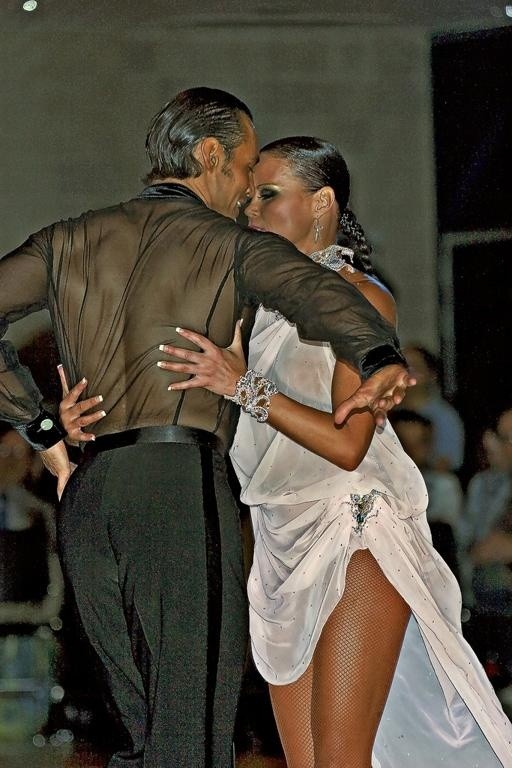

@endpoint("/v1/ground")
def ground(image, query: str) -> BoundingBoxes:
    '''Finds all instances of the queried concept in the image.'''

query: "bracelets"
[225,368,276,424]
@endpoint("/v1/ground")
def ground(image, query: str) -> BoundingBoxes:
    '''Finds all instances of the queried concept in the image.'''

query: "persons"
[1,85,512,768]
[58,135,512,767]
[388,342,512,720]
[0,420,66,635]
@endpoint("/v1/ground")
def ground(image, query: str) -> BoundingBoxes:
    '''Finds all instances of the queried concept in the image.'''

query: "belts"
[83,425,225,456]
